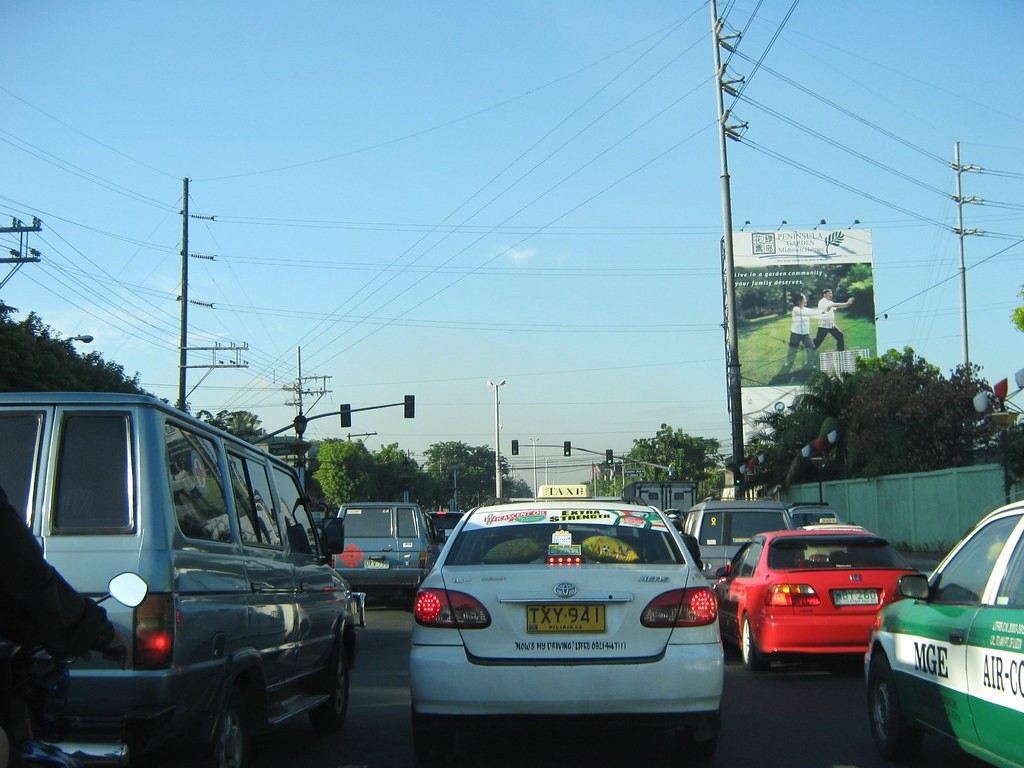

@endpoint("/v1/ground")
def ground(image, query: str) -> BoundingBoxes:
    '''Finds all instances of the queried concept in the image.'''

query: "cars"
[406,484,729,739]
[684,499,1024,768]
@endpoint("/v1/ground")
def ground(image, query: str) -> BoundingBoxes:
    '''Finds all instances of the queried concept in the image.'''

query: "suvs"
[0,390,368,768]
[328,501,466,607]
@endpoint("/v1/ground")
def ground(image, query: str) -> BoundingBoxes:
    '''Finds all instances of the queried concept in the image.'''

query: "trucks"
[624,480,698,515]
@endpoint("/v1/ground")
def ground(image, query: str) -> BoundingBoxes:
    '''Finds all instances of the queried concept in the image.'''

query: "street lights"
[490,381,507,507]
[529,437,540,500]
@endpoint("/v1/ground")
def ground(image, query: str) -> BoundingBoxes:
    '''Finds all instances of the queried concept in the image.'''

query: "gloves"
[45,597,116,661]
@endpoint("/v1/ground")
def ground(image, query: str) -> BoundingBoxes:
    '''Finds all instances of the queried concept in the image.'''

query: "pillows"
[581,536,639,563]
[483,538,542,564]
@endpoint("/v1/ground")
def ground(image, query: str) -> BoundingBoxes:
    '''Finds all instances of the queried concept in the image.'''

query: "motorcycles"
[8,563,149,754]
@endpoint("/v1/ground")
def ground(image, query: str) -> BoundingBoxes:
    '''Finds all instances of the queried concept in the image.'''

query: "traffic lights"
[511,439,519,455]
[606,450,613,464]
[564,442,571,456]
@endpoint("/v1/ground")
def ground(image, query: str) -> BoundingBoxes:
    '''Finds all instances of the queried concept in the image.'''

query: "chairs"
[829,551,845,566]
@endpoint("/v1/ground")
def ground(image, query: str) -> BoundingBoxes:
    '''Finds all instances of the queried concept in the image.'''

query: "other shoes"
[785,361,793,367]
[804,362,817,369]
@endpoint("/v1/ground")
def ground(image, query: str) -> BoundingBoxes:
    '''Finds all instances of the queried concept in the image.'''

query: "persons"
[813,289,855,353]
[0,486,130,768]
[786,294,816,369]
